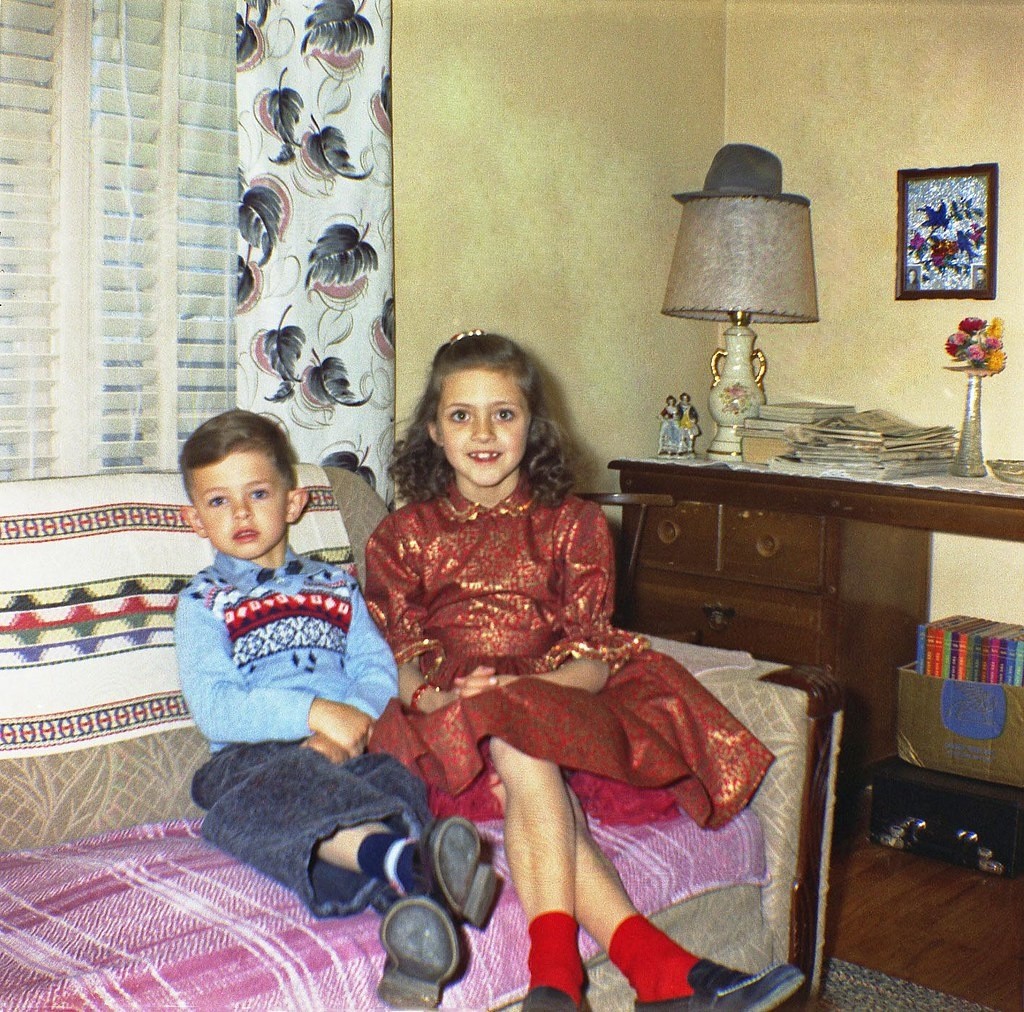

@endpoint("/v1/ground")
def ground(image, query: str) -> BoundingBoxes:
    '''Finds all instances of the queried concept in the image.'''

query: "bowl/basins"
[987,460,1024,484]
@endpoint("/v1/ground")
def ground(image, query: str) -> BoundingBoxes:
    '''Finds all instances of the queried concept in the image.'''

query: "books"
[735,400,960,479]
[916,615,1024,687]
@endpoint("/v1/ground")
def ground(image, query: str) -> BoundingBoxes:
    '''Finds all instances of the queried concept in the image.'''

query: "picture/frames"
[895,164,997,300]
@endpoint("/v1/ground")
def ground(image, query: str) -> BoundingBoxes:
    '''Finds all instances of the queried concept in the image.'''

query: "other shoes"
[634,958,807,1012]
[376,894,462,1012]
[416,815,498,929]
[520,967,589,1012]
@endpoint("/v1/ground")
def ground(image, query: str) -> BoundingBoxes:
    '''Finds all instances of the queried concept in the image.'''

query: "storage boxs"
[895,660,1024,788]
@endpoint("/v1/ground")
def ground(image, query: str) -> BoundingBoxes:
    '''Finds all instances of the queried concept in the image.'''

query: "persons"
[175,409,497,1012]
[658,393,700,455]
[361,330,807,1012]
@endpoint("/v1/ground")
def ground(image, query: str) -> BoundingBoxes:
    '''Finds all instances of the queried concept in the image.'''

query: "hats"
[672,143,811,208]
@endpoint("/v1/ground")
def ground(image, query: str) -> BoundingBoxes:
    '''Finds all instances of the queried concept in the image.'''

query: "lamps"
[662,197,818,459]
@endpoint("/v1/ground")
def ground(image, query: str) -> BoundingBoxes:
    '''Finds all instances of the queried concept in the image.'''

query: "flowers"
[944,317,1006,371]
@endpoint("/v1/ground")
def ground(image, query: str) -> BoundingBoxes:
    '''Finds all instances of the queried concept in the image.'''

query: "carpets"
[820,958,991,1012]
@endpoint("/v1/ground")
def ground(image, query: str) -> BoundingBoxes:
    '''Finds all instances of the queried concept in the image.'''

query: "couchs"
[0,464,845,1012]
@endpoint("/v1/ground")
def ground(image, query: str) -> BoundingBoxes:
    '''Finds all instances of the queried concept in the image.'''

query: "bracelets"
[410,682,437,709]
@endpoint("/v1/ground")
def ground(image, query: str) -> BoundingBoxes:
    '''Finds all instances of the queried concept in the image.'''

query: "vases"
[943,365,992,478]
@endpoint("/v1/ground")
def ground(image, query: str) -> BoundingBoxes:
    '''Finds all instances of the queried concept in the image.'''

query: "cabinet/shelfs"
[608,460,1024,807]
